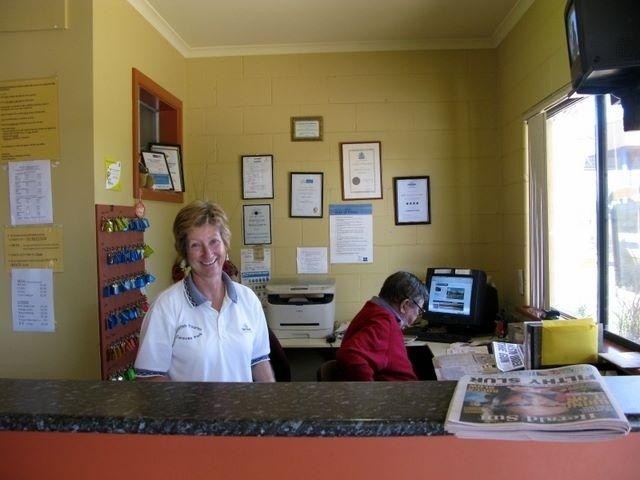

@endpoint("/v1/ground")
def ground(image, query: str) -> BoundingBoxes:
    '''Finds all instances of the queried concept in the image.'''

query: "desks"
[275,325,427,359]
[428,330,524,380]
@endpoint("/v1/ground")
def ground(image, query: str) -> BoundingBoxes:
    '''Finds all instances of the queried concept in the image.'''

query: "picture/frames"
[149,143,185,192]
[290,116,323,142]
[340,141,383,200]
[393,175,432,226]
[142,149,176,190]
[289,171,324,217]
[243,203,271,246]
[240,154,275,200]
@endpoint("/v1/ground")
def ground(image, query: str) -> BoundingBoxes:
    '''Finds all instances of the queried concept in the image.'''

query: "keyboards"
[414,332,468,341]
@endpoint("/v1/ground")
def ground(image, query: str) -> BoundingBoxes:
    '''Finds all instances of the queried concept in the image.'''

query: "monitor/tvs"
[423,267,499,330]
[561,0,640,94]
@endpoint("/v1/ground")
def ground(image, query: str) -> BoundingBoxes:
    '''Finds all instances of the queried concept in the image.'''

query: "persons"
[132,199,276,383]
[222,260,292,383]
[336,270,430,381]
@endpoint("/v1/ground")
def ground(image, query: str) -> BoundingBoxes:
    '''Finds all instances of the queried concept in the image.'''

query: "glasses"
[401,297,426,315]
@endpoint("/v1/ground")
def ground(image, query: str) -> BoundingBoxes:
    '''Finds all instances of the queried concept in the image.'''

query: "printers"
[265,279,336,340]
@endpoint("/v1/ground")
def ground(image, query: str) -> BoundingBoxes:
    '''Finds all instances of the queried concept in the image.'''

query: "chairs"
[316,360,338,382]
